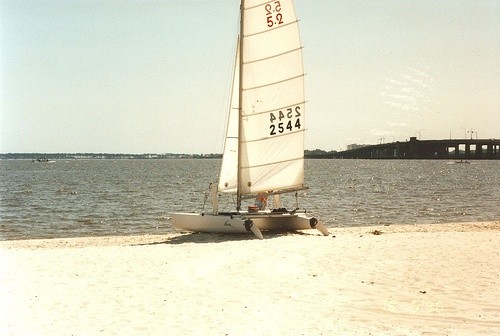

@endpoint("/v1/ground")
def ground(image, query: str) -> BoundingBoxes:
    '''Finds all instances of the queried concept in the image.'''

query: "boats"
[30,158,56,163]
[455,160,470,164]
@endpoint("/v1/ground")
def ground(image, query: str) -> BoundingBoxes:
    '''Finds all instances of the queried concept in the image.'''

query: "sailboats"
[167,0,332,239]
